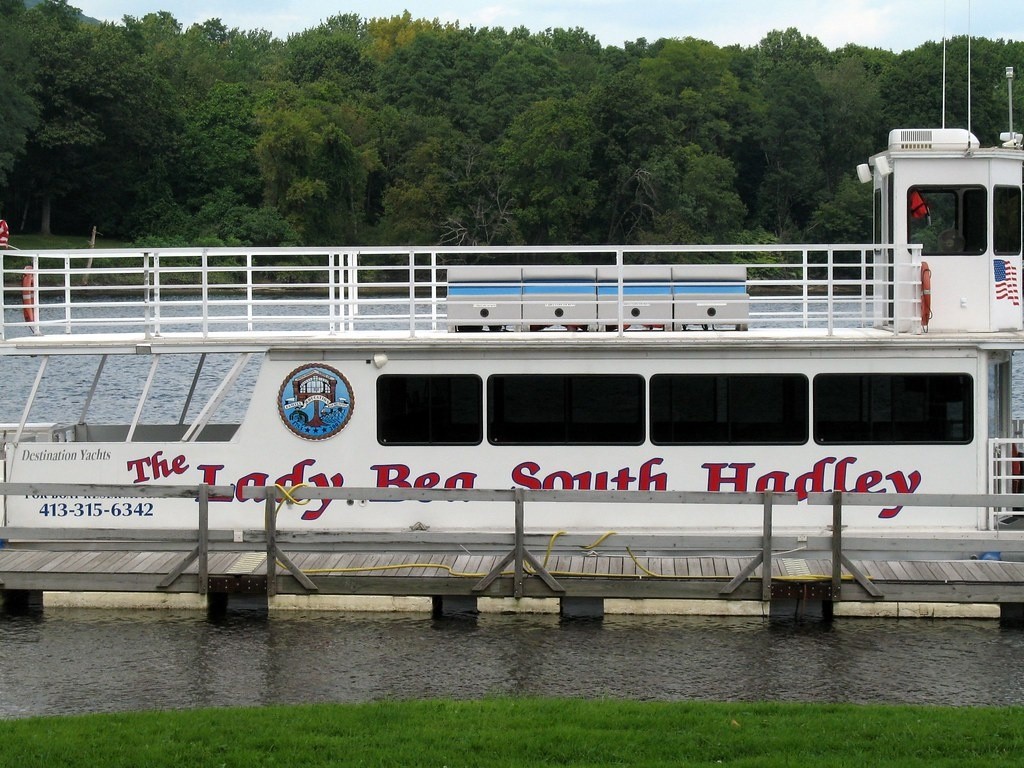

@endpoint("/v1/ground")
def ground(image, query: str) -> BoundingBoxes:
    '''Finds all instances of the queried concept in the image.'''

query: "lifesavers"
[20,265,34,322]
[921,260,931,326]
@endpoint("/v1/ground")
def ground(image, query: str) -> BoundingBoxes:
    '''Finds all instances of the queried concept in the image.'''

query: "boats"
[0,2,1024,559]
